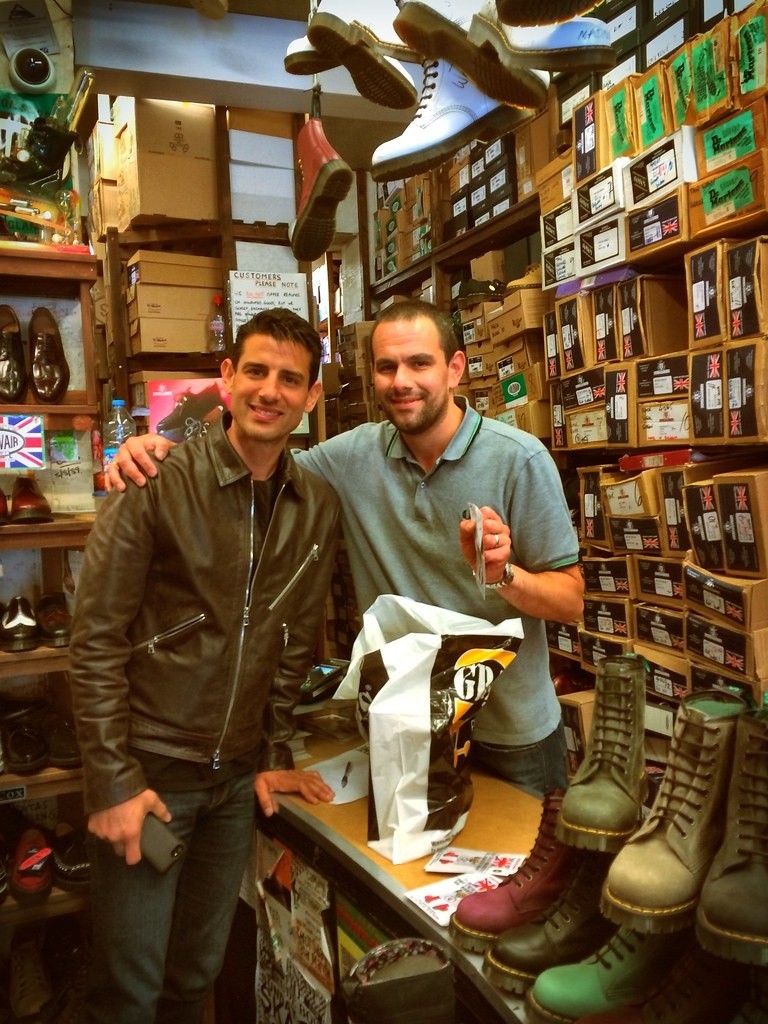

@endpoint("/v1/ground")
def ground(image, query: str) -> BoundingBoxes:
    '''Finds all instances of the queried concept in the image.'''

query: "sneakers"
[10,827,53,901]
[9,928,58,1024]
[0,596,39,651]
[506,262,542,293]
[52,821,93,890]
[457,279,504,308]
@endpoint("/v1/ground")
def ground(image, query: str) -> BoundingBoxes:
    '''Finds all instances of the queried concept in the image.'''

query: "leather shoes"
[29,307,70,405]
[0,486,10,525]
[10,478,53,522]
[37,706,82,766]
[0,700,50,771]
[0,304,28,404]
[284,0,620,109]
[37,592,71,647]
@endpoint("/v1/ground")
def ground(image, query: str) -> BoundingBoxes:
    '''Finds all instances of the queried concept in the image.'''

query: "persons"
[105,300,586,797]
[68,305,351,1024]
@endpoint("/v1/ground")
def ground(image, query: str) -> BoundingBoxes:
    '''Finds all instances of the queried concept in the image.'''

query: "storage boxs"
[0,0,768,765]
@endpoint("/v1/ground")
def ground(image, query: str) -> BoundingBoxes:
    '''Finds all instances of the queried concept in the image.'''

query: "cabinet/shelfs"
[104,103,319,449]
[0,249,106,929]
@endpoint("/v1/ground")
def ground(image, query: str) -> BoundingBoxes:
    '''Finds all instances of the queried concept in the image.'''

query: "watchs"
[472,561,515,589]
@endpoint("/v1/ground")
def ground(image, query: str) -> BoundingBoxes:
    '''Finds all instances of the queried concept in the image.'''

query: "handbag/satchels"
[331,594,525,864]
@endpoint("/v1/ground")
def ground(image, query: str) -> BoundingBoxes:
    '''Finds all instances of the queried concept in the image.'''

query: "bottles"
[104,398,137,481]
[208,294,225,354]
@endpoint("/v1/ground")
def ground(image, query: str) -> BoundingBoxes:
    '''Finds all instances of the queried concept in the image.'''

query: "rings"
[494,533,499,548]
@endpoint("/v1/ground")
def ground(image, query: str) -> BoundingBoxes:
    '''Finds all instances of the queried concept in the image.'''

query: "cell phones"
[136,814,188,877]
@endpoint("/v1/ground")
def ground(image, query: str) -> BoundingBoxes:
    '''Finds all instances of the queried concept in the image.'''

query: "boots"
[291,119,354,262]
[0,117,78,200]
[370,57,538,182]
[449,786,768,1024]
[556,656,768,966]
[344,937,455,1024]
